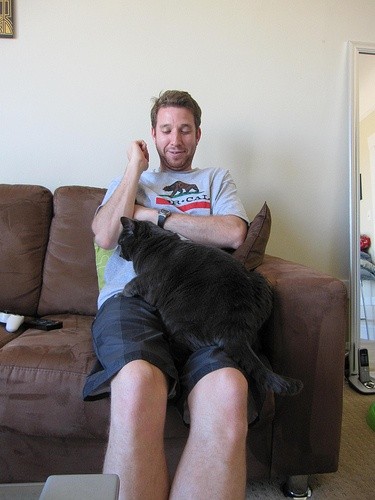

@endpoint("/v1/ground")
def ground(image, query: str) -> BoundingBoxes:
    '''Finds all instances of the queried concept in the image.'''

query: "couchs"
[0,184,348,500]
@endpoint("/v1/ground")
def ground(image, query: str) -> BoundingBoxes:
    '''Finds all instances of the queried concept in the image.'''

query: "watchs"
[157,208,172,228]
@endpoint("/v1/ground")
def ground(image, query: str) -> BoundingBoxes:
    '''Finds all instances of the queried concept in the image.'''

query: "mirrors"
[348,40,375,376]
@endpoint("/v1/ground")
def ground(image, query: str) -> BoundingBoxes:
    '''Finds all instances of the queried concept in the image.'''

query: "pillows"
[233,201,272,272]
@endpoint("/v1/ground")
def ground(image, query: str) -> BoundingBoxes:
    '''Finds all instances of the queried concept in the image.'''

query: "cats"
[118,217,306,397]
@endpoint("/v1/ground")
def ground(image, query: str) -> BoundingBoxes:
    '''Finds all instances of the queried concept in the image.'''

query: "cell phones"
[22,318,62,332]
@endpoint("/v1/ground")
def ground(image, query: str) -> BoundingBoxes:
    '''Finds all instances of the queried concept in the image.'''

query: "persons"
[81,90,250,500]
